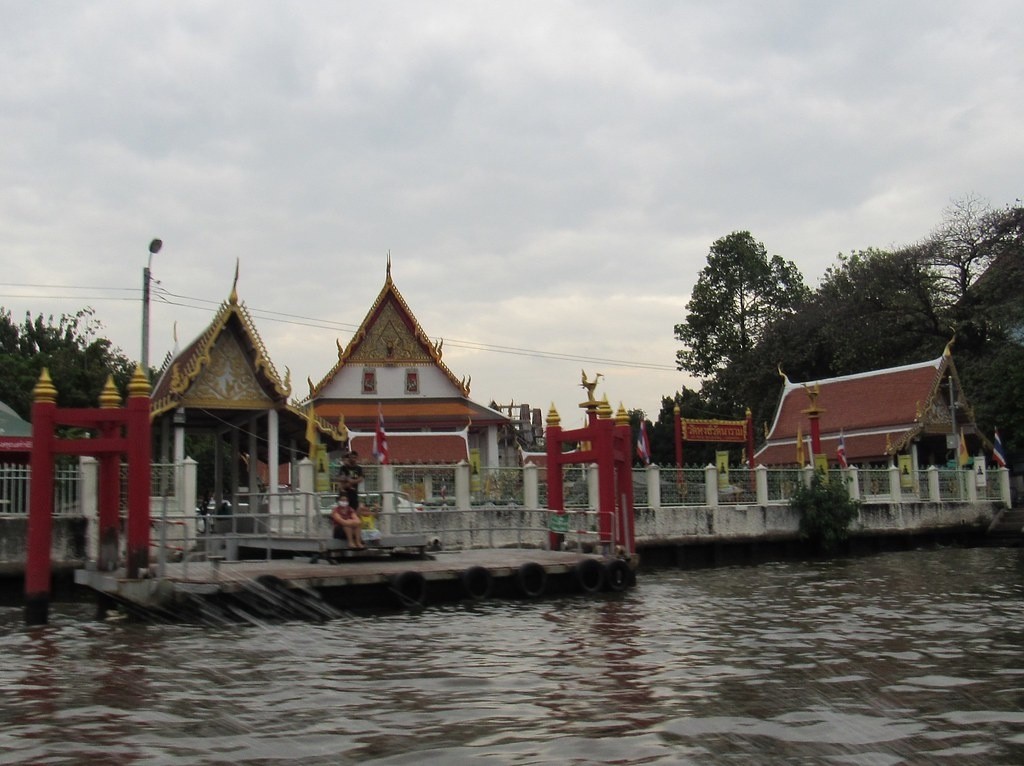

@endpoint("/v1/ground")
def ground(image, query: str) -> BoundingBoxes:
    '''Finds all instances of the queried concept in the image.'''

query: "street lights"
[141,239,163,378]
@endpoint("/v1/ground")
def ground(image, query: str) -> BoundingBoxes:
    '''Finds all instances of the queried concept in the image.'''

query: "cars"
[329,493,424,513]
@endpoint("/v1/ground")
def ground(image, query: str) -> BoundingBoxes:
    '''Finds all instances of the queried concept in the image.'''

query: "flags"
[796,425,804,463]
[441,477,447,498]
[636,416,652,465]
[837,432,847,468]
[959,430,969,465]
[993,428,1006,466]
[373,406,390,464]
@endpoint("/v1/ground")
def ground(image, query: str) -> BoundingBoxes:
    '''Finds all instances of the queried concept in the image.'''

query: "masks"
[338,501,347,505]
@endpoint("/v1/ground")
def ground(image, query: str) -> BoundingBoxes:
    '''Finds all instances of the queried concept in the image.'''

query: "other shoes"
[348,544,364,548]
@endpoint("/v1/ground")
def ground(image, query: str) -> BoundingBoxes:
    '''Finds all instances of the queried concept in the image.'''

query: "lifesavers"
[247,574,291,617]
[515,561,548,598]
[463,566,492,601]
[390,570,428,608]
[575,558,605,594]
[606,558,630,592]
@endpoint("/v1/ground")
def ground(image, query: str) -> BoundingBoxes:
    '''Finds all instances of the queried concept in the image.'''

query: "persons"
[331,450,374,548]
